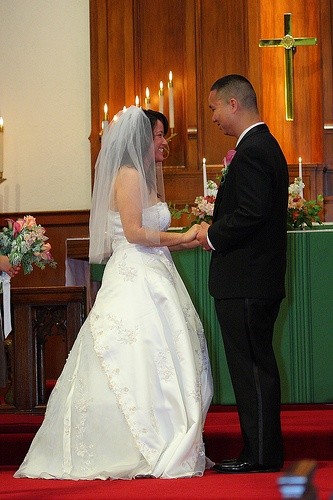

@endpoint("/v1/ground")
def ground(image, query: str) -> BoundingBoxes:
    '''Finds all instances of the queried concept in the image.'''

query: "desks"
[65,226,333,405]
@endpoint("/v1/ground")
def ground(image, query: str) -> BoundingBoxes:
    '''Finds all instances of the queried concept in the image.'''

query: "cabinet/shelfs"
[88,0,333,222]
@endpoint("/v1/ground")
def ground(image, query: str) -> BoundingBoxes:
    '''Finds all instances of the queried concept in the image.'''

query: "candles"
[157,81,164,115]
[202,157,208,197]
[144,86,151,110]
[135,96,140,108]
[168,70,175,129]
[299,156,303,199]
[102,103,109,128]
[0,118,5,179]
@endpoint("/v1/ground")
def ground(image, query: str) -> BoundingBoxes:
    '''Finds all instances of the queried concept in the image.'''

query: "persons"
[0,255,21,278]
[13,105,214,481]
[196,74,289,473]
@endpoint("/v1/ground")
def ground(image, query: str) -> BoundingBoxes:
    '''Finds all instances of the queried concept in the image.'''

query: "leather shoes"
[212,458,281,472]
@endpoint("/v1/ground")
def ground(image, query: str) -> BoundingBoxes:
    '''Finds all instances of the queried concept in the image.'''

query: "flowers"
[286,177,326,230]
[167,150,236,233]
[0,215,58,293]
